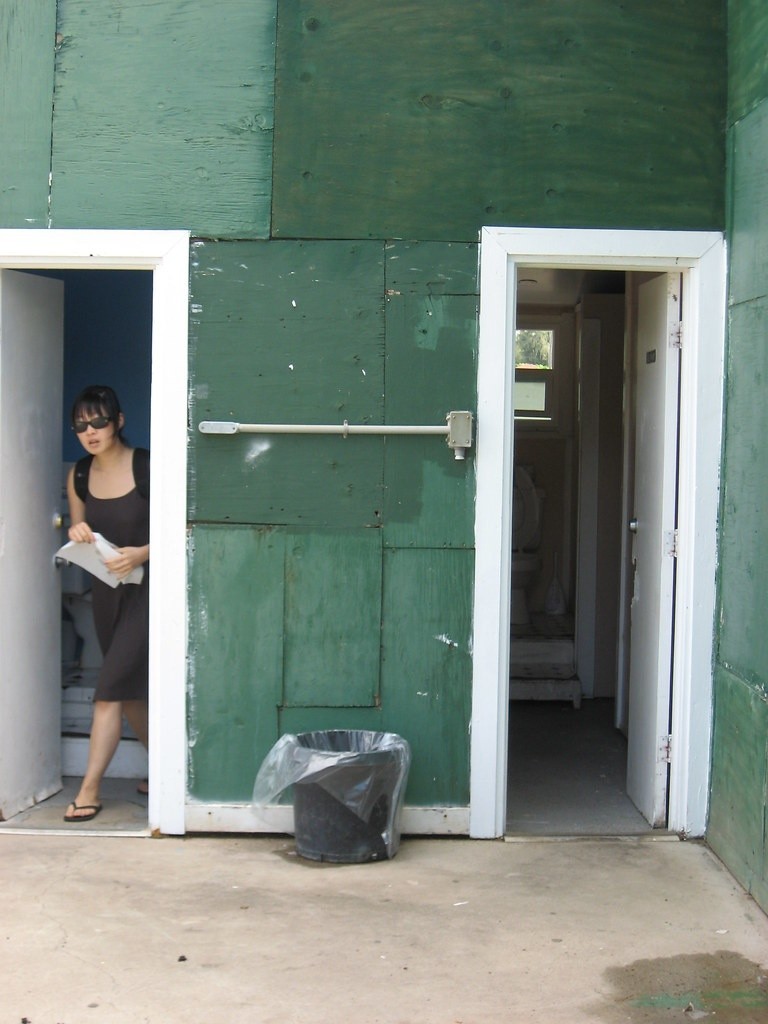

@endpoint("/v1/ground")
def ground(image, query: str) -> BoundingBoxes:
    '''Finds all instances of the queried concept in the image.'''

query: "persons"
[62,384,150,823]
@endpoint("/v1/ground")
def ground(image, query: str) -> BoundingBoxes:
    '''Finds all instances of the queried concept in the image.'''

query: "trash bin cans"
[289,729,407,864]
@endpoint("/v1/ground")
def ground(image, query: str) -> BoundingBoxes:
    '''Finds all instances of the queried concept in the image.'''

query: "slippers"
[137,778,148,795]
[63,802,103,822]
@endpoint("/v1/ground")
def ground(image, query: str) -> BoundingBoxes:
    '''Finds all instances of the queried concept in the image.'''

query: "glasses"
[71,416,114,433]
